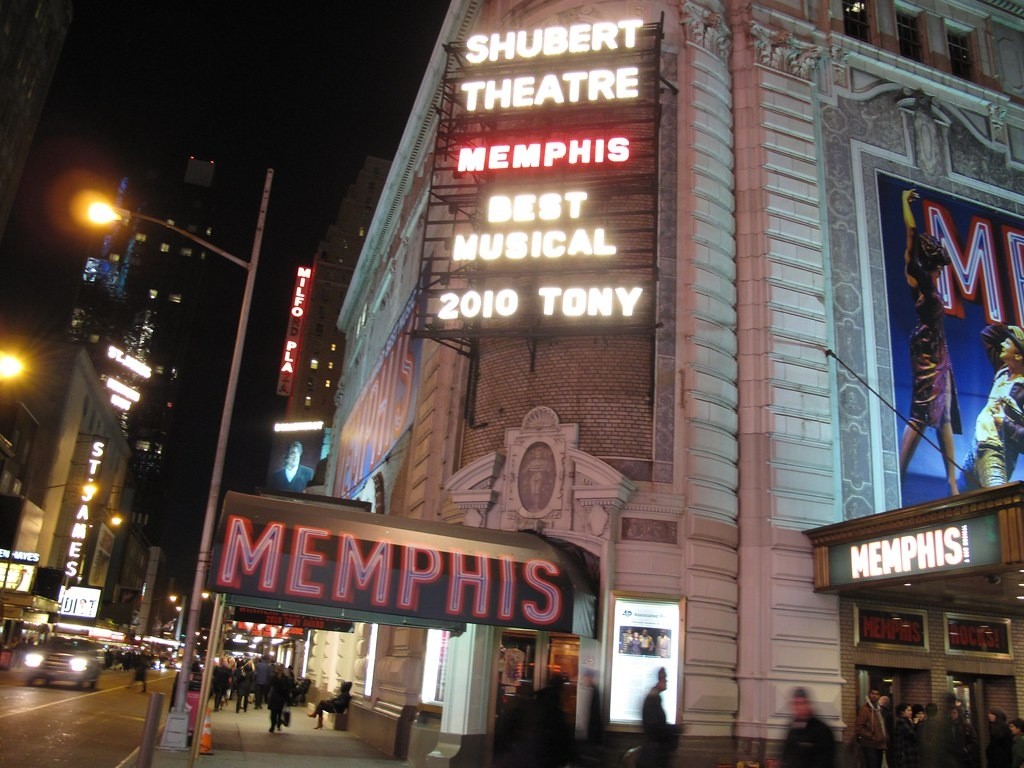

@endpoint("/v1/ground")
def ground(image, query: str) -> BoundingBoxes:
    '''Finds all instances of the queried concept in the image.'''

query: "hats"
[1008,326,1024,350]
[990,705,1008,720]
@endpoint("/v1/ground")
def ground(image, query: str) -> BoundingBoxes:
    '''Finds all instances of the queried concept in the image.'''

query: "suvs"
[24,638,100,690]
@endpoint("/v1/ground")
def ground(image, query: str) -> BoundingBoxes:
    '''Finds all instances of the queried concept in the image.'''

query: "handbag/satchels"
[283,707,290,727]
[237,670,246,682]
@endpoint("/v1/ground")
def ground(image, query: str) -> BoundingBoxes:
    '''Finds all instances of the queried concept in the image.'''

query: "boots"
[307,710,318,718]
[314,716,323,729]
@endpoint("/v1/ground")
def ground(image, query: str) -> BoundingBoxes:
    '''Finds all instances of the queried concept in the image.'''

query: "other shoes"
[277,725,280,731]
[269,728,274,732]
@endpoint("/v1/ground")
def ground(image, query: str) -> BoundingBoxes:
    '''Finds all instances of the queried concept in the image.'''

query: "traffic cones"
[200,708,216,753]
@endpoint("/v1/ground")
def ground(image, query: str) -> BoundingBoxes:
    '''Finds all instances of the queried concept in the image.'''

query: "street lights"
[68,169,280,748]
[168,592,186,641]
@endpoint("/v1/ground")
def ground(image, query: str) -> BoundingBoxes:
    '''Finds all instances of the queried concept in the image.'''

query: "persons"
[780,687,1024,768]
[271,441,314,493]
[0,637,355,733]
[619,628,671,658]
[899,189,1024,498]
[487,667,686,768]
